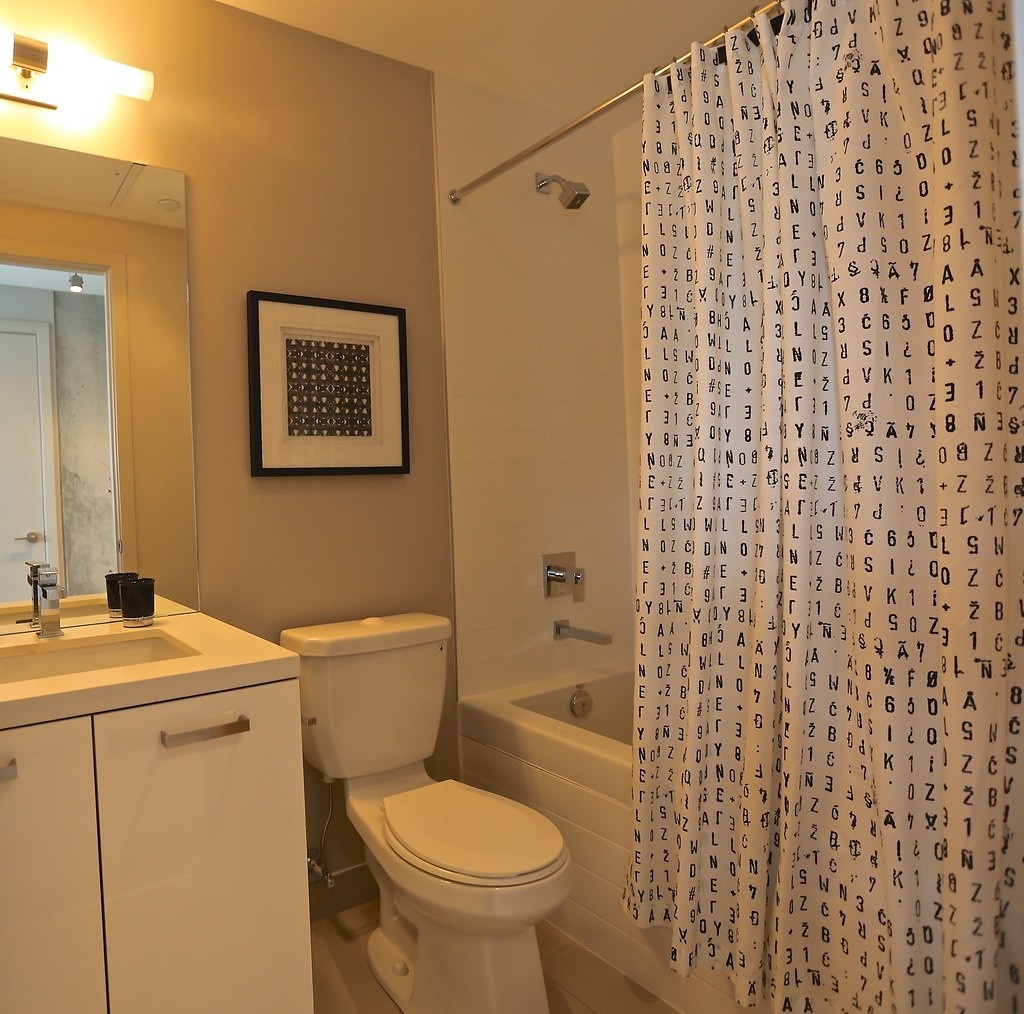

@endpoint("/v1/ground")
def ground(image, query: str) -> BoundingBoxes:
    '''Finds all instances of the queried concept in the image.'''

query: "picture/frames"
[246,289,411,476]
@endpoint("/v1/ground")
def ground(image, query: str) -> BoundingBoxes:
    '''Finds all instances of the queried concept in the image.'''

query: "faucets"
[36,568,66,639]
[552,615,614,649]
[23,561,51,627]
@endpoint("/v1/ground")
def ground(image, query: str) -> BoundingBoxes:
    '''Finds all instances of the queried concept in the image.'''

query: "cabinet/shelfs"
[1,654,317,1012]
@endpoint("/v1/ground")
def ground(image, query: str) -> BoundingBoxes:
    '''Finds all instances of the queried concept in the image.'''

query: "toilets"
[278,611,576,1013]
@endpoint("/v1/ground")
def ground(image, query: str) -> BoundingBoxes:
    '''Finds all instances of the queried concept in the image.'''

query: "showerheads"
[536,171,588,212]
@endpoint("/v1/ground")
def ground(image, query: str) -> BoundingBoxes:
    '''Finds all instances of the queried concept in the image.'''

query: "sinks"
[0,626,205,691]
[1,595,118,626]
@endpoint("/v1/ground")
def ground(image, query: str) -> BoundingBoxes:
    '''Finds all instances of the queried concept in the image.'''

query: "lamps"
[0,24,154,112]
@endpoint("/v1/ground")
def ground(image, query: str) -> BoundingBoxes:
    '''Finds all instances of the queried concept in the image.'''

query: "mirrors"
[0,264,120,603]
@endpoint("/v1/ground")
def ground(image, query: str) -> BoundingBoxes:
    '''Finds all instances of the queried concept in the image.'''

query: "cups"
[118,577,155,628]
[105,572,139,618]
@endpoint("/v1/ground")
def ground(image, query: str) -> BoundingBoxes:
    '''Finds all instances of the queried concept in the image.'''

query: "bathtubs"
[460,666,778,1012]
